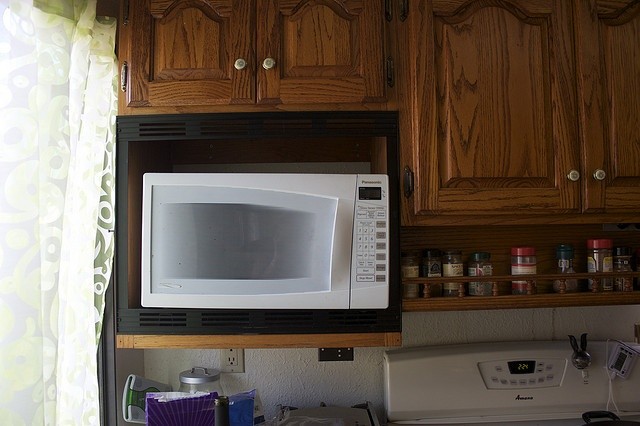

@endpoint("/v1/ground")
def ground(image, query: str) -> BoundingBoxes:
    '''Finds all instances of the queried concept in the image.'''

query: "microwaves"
[141,172,389,311]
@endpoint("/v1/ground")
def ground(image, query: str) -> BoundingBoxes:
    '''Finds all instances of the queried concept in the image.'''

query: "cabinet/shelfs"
[399,3,639,226]
[116,0,399,116]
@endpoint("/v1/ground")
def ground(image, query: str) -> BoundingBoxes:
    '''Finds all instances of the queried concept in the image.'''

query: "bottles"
[177,365,225,397]
[586,238,615,293]
[421,247,444,299]
[510,246,538,296]
[399,256,421,298]
[613,246,634,294]
[468,252,493,296]
[551,241,578,293]
[439,248,467,298]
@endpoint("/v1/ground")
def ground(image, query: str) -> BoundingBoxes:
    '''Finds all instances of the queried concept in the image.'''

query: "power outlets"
[219,348,245,373]
